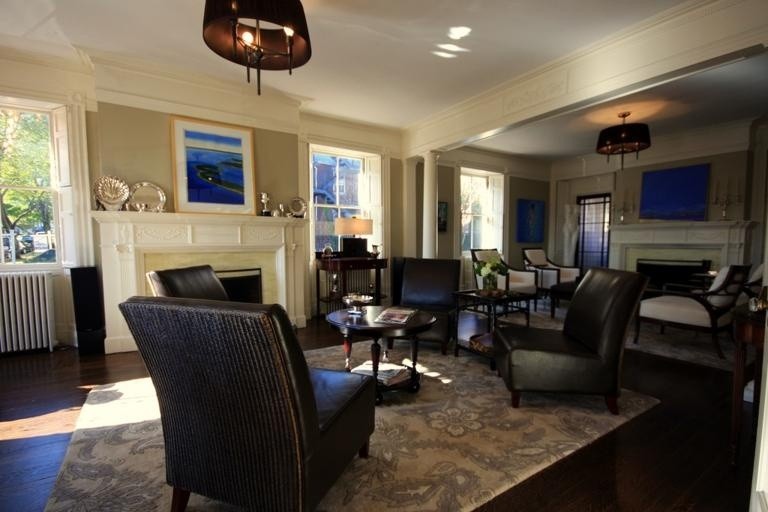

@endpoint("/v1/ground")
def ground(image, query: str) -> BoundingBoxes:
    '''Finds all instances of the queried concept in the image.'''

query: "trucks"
[313,189,357,251]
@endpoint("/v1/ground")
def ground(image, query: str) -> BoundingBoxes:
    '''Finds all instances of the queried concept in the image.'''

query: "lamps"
[596,112,651,172]
[203,1,312,95]
[333,216,373,256]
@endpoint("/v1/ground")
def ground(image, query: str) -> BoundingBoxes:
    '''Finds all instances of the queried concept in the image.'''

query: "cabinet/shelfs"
[315,257,387,330]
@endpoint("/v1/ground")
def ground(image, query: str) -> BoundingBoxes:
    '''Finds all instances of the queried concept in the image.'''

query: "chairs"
[470,247,581,311]
[489,269,650,415]
[388,257,461,356]
[145,262,229,301]
[118,294,379,511]
[633,261,765,360]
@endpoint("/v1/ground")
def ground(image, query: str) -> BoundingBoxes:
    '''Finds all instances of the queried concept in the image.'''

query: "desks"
[728,301,768,466]
[456,290,532,334]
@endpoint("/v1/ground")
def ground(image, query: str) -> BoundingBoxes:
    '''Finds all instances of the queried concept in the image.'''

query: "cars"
[2,226,46,258]
[25,249,56,262]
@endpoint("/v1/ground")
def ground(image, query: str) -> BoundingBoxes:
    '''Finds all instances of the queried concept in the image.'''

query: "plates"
[288,196,307,216]
[128,181,166,213]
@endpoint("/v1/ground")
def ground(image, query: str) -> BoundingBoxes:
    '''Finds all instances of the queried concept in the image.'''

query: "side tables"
[550,281,578,318]
[461,288,542,333]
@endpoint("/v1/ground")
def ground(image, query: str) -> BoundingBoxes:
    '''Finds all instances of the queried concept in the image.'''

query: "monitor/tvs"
[639,162,711,223]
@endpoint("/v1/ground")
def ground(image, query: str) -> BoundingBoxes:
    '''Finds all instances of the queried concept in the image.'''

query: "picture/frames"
[438,201,448,232]
[170,115,257,216]
[515,198,544,244]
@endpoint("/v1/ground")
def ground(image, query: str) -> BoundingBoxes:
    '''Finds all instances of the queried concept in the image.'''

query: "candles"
[612,187,636,210]
[712,174,739,204]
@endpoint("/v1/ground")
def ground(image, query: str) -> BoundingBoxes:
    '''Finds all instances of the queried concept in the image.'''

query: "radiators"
[0,272,59,353]
[319,268,373,315]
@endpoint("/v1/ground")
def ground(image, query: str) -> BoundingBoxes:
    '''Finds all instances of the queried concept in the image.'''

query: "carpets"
[461,295,757,373]
[42,333,661,511]
